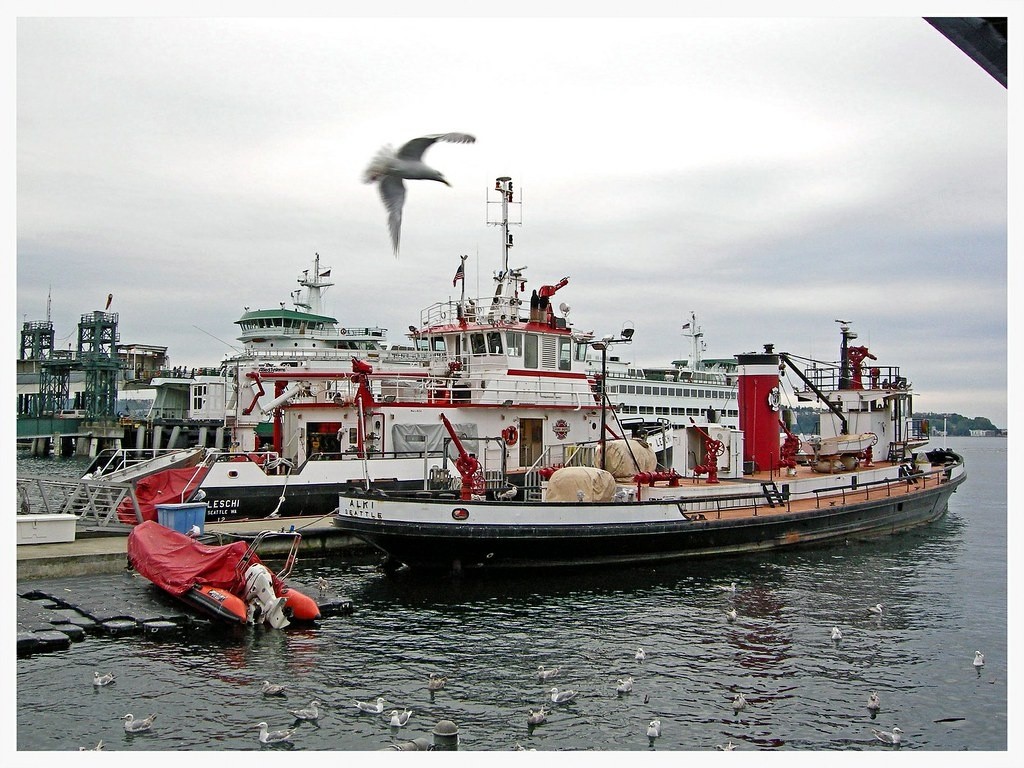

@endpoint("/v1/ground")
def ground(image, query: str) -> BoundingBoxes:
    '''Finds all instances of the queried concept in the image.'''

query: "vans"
[53,407,96,420]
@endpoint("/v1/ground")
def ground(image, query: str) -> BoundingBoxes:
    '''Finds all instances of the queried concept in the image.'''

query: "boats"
[128,520,321,624]
[333,344,968,574]
[76,176,786,527]
[801,434,876,455]
[144,250,739,430]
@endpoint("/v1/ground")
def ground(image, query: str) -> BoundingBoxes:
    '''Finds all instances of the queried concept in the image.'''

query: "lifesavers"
[506,426,518,444]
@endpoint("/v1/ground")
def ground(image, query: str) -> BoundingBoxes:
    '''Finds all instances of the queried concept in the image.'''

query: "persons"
[132,366,218,379]
[307,432,327,461]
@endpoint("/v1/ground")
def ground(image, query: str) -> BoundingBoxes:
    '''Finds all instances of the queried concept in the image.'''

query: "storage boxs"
[156,502,208,537]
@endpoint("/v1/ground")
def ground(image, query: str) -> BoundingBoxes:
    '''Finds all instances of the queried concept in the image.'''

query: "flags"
[453,264,465,287]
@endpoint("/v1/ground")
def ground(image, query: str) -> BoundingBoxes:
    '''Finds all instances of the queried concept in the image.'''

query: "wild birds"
[259,680,286,695]
[286,700,323,720]
[78,739,106,751]
[388,707,413,727]
[92,670,117,686]
[644,693,650,704]
[722,608,737,622]
[635,648,646,659]
[867,690,880,709]
[120,712,157,733]
[362,130,477,262]
[427,675,447,689]
[867,603,884,613]
[646,719,661,736]
[253,721,298,744]
[871,727,904,744]
[972,650,985,666]
[716,740,740,751]
[192,490,207,502]
[537,665,560,679]
[184,525,201,540]
[528,704,545,725]
[549,688,579,702]
[731,692,749,709]
[91,466,102,481]
[830,627,843,640]
[355,697,387,714]
[617,676,634,692]
